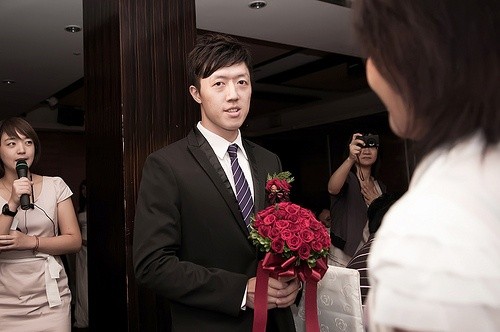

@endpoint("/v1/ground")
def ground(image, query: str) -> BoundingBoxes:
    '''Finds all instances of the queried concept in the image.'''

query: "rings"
[275,298,278,305]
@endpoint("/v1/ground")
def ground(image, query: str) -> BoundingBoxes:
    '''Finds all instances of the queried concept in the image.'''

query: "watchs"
[2,204,18,217]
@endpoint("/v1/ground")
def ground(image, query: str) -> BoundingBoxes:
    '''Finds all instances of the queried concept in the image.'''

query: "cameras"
[357,135,379,148]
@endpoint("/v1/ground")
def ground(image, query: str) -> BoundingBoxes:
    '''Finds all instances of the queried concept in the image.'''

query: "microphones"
[16,159,30,210]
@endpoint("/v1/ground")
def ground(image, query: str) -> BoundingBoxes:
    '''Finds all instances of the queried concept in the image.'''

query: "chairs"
[290,265,365,332]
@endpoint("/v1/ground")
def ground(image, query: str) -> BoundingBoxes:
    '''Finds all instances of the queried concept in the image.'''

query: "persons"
[353,0,500,332]
[131,32,301,332]
[344,194,401,307]
[316,208,332,229]
[327,127,388,266]
[0,116,83,332]
[73,210,89,328]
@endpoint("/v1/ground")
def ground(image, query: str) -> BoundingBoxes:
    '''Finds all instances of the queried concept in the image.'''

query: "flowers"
[265,171,294,205]
[247,202,331,262]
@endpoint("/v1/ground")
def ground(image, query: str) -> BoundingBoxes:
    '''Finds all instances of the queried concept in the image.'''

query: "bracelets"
[34,235,39,250]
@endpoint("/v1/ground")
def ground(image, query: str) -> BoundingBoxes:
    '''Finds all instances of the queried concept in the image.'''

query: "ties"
[228,144,255,232]
